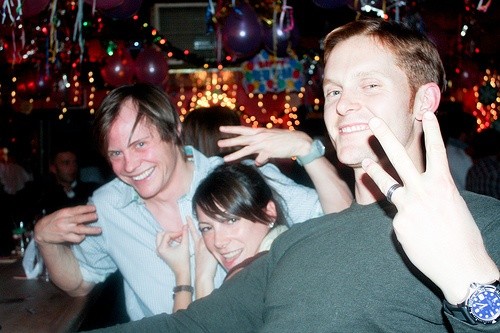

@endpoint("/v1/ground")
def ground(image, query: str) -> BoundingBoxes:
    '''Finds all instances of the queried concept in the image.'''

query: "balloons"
[0,0,299,86]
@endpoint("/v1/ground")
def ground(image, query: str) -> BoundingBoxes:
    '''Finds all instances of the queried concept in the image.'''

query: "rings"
[387,183,402,204]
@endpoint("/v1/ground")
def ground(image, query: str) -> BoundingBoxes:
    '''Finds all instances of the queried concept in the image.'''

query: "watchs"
[296,140,326,166]
[172,285,194,298]
[445,281,500,325]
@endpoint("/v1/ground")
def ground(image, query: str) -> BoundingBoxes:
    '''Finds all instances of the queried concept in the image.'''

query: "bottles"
[12,208,47,261]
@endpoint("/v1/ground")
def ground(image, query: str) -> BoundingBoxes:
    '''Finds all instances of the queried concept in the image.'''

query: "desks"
[0,254,109,333]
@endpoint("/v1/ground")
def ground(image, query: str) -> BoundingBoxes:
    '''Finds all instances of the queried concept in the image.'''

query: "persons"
[0,82,500,322]
[79,21,500,333]
